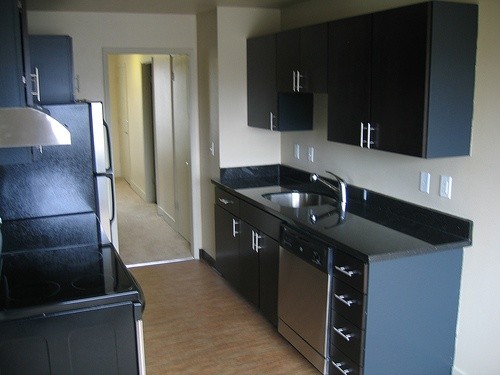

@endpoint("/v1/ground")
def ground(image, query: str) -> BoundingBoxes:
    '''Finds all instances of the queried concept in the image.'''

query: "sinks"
[261,191,333,209]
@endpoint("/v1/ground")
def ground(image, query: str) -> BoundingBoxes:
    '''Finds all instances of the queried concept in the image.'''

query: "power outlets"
[307,147,313,163]
[440,176,453,199]
[294,144,299,159]
[419,172,430,194]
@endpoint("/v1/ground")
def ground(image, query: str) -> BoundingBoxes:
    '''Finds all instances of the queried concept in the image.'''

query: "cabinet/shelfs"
[247,0,480,160]
[0,0,74,165]
[213,186,463,375]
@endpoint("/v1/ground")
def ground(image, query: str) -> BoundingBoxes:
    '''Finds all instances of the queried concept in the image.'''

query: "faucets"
[310,170,347,203]
[310,203,347,229]
